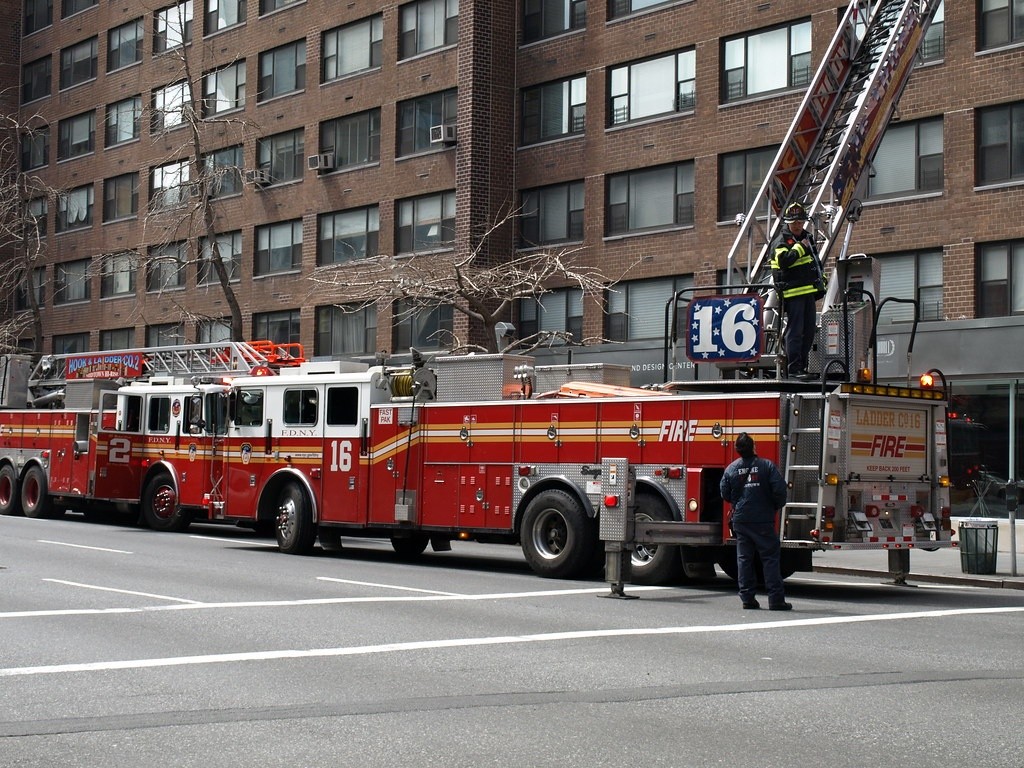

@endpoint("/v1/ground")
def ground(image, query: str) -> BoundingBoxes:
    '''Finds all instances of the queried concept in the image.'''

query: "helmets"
[783,202,806,223]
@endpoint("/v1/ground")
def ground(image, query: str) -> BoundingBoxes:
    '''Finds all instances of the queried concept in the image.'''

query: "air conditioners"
[430,125,457,143]
[245,169,269,184]
[189,182,212,197]
[308,153,333,170]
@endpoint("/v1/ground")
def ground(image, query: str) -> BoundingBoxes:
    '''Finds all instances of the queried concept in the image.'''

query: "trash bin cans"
[958,520,998,576]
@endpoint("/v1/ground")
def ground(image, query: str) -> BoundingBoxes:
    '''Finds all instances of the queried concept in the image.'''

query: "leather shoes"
[788,368,820,381]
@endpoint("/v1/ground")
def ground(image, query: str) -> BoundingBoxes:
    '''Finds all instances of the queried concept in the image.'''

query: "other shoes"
[770,601,792,611]
[743,600,759,609]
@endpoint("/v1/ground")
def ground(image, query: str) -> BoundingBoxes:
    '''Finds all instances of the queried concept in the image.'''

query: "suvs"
[948,449,998,490]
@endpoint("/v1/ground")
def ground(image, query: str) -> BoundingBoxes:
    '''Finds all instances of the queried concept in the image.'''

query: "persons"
[720,432,793,610]
[770,201,828,380]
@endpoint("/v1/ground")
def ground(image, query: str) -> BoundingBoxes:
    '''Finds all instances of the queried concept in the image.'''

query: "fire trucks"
[948,413,991,453]
[1,0,956,585]
